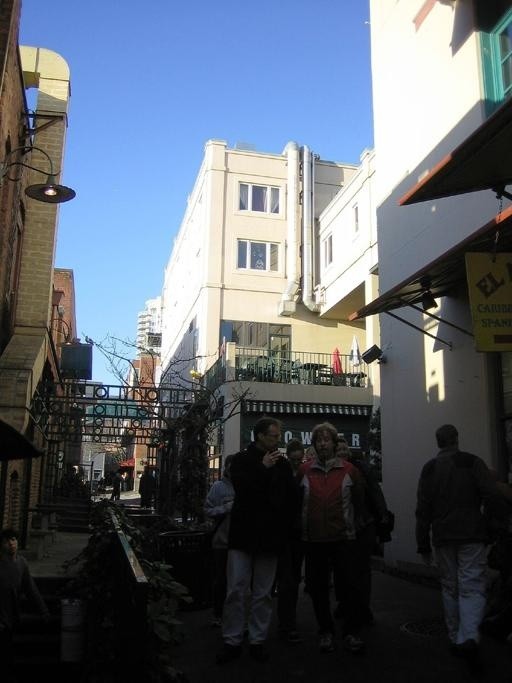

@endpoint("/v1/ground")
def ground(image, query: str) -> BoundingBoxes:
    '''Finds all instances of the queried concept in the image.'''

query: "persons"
[87,471,123,502]
[200,416,388,660]
[1,528,31,683]
[415,422,503,656]
[140,466,156,507]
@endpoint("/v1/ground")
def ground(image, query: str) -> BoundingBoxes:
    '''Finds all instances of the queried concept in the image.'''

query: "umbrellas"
[331,347,343,373]
[348,335,363,373]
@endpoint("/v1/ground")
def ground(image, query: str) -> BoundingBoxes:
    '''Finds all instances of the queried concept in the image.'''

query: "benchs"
[318,372,367,387]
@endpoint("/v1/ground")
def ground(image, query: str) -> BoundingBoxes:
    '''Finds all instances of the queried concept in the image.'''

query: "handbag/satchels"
[199,530,217,559]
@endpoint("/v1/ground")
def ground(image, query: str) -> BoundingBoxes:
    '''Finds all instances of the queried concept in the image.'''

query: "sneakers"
[248,644,272,663]
[214,643,243,665]
[278,629,302,645]
[318,631,338,655]
[447,638,485,678]
[340,634,367,654]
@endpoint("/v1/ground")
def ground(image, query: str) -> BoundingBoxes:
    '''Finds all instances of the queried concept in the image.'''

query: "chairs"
[26,511,56,561]
[239,355,300,387]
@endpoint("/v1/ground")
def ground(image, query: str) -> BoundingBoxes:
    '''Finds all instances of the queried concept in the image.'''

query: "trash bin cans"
[157,529,211,612]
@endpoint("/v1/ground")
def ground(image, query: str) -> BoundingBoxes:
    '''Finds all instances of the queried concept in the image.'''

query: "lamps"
[361,345,385,366]
[0,145,76,206]
[421,291,438,310]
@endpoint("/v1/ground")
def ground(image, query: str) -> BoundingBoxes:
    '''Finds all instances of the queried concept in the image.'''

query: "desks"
[293,362,328,383]
